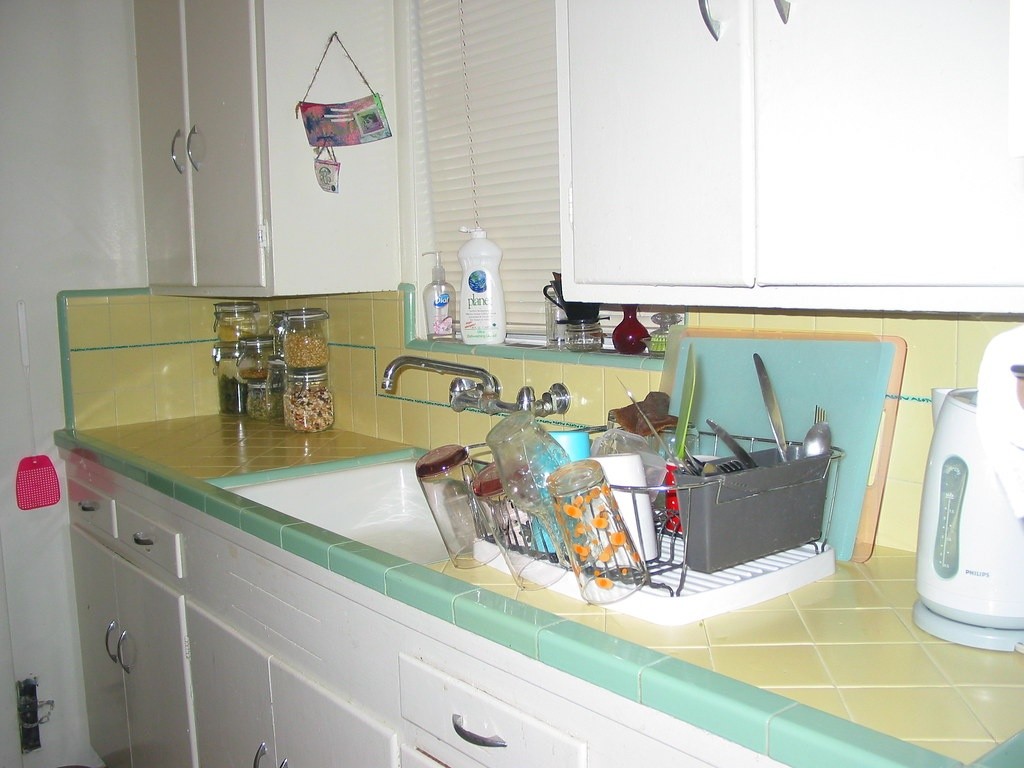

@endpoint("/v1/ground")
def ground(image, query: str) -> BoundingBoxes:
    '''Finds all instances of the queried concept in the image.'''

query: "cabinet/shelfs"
[134,0,402,298]
[552,0,1024,319]
[186,520,717,768]
[460,426,846,596]
[65,461,200,768]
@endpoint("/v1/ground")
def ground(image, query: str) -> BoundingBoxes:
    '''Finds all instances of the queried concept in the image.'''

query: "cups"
[529,431,590,553]
[589,453,657,568]
[472,461,570,591]
[547,460,648,606]
[486,411,601,571]
[415,445,501,568]
[543,281,600,319]
[544,290,569,349]
[665,456,720,535]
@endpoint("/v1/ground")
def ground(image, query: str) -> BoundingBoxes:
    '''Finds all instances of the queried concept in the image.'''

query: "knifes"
[674,345,696,461]
[706,419,756,470]
[753,354,789,463]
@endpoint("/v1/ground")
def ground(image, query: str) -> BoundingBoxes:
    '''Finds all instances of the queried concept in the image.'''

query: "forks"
[815,405,826,425]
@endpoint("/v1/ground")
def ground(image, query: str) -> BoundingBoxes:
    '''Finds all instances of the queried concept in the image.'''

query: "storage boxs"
[674,444,835,574]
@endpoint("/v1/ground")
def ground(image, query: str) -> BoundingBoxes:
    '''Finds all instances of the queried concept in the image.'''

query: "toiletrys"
[422,251,458,341]
[456,226,507,347]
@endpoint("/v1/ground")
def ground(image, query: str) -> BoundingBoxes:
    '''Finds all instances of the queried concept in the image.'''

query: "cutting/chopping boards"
[649,325,908,562]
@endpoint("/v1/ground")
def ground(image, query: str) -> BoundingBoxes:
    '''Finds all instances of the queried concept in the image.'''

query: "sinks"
[203,446,488,565]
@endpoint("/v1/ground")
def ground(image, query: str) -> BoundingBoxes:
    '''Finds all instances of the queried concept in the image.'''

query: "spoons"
[803,422,831,458]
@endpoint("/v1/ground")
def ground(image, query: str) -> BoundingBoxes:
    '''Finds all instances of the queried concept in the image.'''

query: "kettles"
[913,385,1024,656]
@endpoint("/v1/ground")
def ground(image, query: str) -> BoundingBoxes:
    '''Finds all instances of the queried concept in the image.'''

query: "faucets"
[382,357,573,421]
